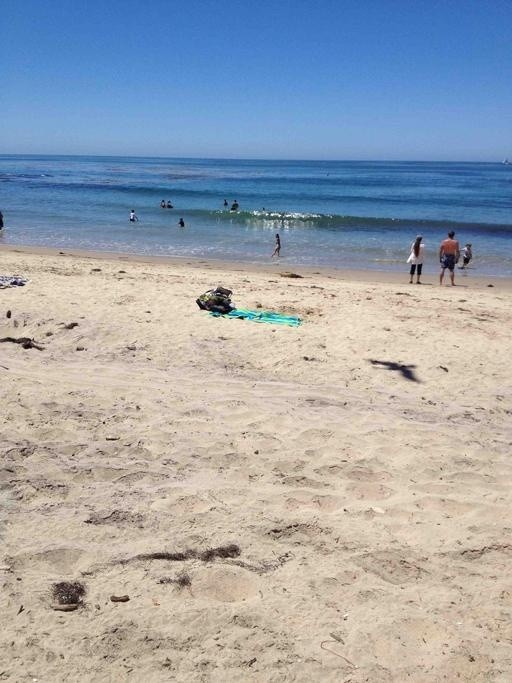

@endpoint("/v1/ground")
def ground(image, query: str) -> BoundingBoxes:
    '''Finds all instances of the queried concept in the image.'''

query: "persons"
[440,230,461,285]
[224,199,228,206]
[177,217,184,227]
[271,234,281,256]
[167,199,174,208]
[232,199,239,208]
[159,199,167,209]
[406,235,426,283]
[458,243,473,269]
[129,209,139,221]
